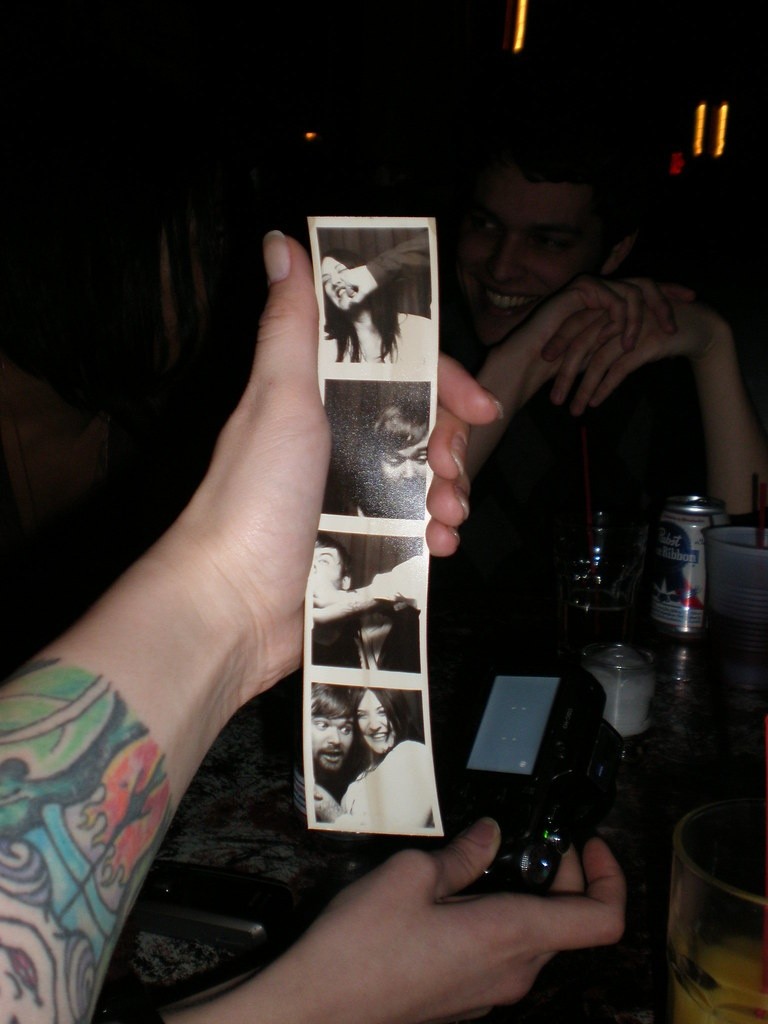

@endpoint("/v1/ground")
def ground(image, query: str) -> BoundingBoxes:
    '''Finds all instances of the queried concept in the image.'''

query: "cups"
[700,524,768,690]
[555,522,650,664]
[666,799,768,1024]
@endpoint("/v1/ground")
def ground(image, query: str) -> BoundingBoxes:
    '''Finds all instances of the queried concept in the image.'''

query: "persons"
[347,402,426,516]
[0,229,631,1023]
[312,684,432,829]
[319,230,430,364]
[435,114,767,580]
[312,534,421,674]
[0,107,224,553]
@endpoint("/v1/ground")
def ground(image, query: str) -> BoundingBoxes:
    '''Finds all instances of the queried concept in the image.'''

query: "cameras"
[434,659,626,897]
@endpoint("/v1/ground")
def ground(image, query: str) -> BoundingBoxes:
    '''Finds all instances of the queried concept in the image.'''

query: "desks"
[92,568,768,1024]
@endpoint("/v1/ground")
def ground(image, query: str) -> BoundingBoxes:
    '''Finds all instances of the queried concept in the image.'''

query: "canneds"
[650,494,729,632]
